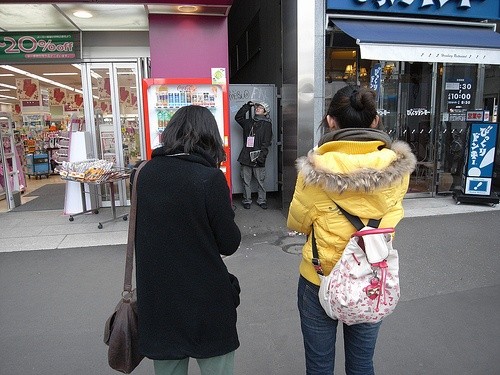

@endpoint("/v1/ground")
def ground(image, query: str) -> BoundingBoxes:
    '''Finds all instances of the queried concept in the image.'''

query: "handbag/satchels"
[103,287,145,374]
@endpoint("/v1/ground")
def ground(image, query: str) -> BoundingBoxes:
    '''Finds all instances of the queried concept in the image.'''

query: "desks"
[60,175,130,228]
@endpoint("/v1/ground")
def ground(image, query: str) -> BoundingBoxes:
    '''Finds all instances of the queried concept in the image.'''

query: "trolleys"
[25,150,49,180]
[58,158,132,229]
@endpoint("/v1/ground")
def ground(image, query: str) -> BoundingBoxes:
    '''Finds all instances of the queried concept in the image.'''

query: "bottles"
[159,130,162,143]
[157,109,178,129]
[158,87,216,104]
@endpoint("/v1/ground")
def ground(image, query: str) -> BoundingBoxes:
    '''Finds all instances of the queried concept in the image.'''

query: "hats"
[255,102,271,112]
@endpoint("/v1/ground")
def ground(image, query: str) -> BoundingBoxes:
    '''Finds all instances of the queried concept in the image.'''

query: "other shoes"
[244,204,250,209]
[257,203,268,209]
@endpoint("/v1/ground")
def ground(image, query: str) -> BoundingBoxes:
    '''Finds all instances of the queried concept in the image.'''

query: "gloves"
[246,100,254,108]
[256,157,265,165]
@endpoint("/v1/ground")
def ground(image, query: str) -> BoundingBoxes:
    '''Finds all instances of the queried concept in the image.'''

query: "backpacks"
[312,201,400,326]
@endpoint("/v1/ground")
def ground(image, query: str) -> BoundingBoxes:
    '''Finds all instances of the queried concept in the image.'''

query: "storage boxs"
[25,154,51,174]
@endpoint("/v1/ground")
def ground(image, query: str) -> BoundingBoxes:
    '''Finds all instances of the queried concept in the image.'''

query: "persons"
[286,84,418,375]
[234,101,273,210]
[128,105,241,375]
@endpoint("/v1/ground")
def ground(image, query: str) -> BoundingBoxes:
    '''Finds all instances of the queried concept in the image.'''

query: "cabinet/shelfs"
[99,124,140,175]
